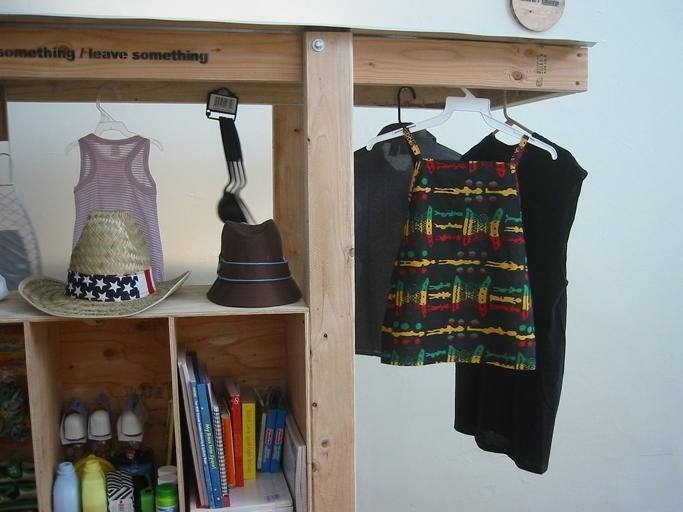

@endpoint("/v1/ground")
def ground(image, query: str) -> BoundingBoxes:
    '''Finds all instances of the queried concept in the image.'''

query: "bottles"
[154,465,178,512]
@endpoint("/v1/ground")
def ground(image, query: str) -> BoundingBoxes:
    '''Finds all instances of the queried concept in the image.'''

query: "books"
[176,341,306,512]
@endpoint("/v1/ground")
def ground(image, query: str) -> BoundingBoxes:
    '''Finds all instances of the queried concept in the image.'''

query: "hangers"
[353,84,574,170]
[62,86,164,155]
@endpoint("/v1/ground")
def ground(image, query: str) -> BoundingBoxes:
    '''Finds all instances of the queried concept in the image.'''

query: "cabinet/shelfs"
[0,0,589,512]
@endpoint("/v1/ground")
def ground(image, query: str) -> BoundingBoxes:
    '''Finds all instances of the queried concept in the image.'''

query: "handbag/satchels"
[59,399,87,447]
[87,393,113,441]
[117,393,148,442]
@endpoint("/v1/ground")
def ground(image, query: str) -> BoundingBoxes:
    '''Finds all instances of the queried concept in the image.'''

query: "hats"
[17,210,191,320]
[207,219,302,307]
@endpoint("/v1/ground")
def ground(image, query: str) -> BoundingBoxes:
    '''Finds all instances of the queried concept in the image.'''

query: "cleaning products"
[53,461,82,512]
[81,453,109,512]
